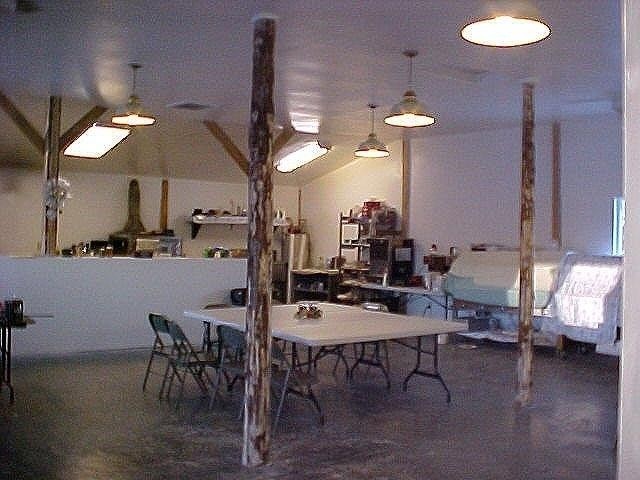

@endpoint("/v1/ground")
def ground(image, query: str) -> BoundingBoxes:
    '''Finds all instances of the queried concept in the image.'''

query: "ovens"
[109,231,182,258]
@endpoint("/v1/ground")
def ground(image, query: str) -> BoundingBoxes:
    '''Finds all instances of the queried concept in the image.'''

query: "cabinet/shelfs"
[338,209,400,305]
[290,268,337,303]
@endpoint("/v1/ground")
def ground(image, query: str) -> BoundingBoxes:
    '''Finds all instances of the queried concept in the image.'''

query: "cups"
[3,295,24,320]
[464,339,472,350]
[195,199,247,216]
[423,272,441,293]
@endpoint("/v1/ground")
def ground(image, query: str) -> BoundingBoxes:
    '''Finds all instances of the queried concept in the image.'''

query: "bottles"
[272,208,289,225]
[381,264,392,287]
[317,255,324,271]
[449,246,456,257]
[489,320,498,332]
[461,319,472,331]
[73,240,114,257]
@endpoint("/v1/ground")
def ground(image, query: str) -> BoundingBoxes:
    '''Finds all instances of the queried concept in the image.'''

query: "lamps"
[460,1,551,48]
[383,49,437,130]
[110,62,157,128]
[274,140,330,173]
[354,103,390,159]
[63,122,132,160]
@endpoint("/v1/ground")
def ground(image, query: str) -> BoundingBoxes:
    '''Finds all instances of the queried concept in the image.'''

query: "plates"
[458,344,478,349]
[427,247,441,255]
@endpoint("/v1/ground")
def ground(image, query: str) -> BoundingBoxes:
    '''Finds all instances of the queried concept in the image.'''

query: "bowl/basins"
[62,249,72,256]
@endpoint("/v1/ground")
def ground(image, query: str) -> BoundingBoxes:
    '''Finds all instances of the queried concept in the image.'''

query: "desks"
[1,302,54,405]
[341,279,456,345]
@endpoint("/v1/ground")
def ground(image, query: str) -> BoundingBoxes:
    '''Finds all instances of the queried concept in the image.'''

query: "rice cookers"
[230,287,248,306]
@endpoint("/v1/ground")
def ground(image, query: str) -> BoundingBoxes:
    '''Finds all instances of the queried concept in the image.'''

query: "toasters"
[207,248,231,257]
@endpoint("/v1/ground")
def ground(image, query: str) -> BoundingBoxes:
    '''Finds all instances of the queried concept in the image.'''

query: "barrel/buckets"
[422,243,448,289]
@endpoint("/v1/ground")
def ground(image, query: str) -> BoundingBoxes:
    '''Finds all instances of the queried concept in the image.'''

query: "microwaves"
[367,237,414,277]
